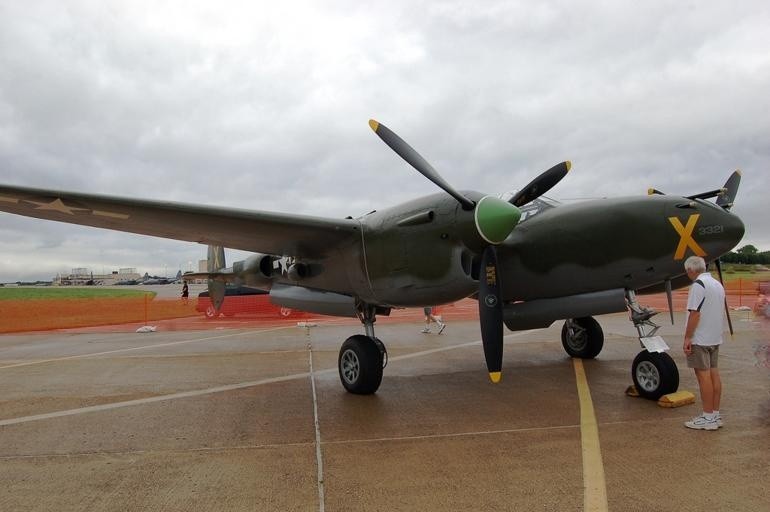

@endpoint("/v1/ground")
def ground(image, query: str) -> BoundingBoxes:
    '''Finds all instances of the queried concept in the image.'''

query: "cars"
[196,283,314,319]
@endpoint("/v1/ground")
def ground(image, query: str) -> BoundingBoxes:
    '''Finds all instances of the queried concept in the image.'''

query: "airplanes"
[0,279,178,287]
[0,119,745,400]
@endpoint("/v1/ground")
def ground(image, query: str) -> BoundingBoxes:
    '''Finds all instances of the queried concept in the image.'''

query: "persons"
[682,255,726,431]
[180,280,189,306]
[420,307,445,335]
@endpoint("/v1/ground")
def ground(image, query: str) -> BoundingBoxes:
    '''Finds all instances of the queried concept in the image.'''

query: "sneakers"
[684,415,724,432]
